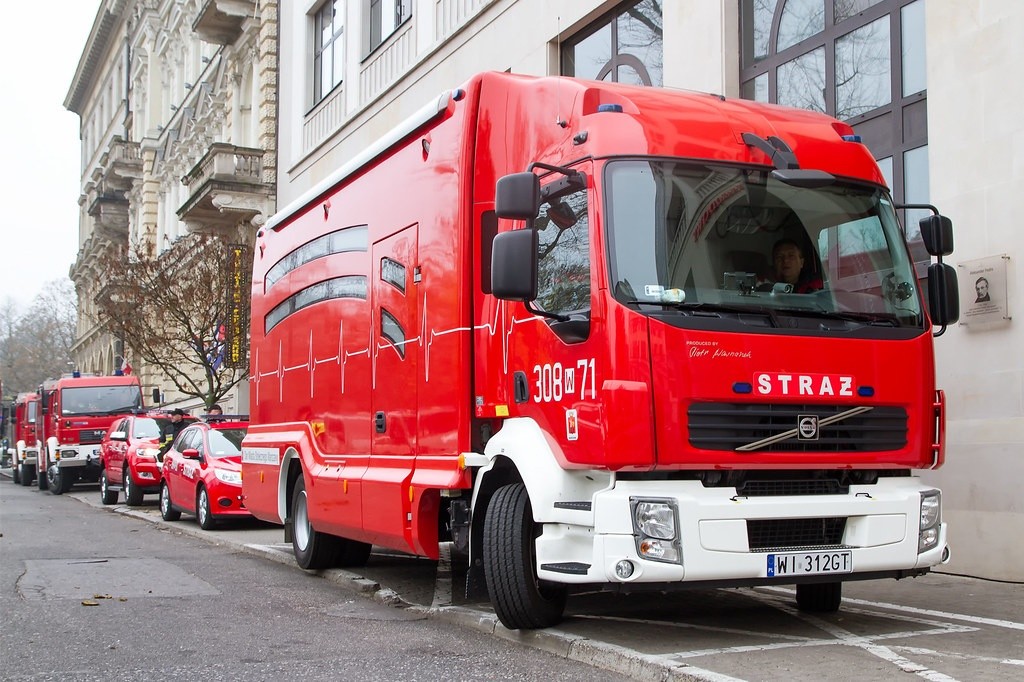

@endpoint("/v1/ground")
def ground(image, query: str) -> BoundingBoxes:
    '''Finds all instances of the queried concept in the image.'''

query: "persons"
[974,277,990,303]
[205,405,226,424]
[157,408,189,462]
[756,237,823,294]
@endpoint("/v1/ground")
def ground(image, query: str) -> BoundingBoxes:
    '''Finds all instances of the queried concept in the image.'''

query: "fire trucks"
[34,360,164,496]
[237,68,960,630]
[7,384,46,487]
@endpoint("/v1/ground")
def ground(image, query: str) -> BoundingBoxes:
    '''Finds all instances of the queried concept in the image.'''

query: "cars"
[159,414,260,530]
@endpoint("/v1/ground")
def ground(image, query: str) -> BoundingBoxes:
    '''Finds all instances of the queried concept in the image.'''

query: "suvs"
[99,407,204,506]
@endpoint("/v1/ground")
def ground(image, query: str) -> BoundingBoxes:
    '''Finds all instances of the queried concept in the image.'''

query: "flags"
[192,320,225,374]
[121,360,133,375]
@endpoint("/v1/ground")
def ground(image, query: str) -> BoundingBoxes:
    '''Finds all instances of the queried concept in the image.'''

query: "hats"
[203,405,221,411]
[170,408,184,415]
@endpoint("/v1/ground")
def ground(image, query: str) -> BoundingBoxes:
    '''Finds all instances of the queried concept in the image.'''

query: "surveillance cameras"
[774,282,794,293]
